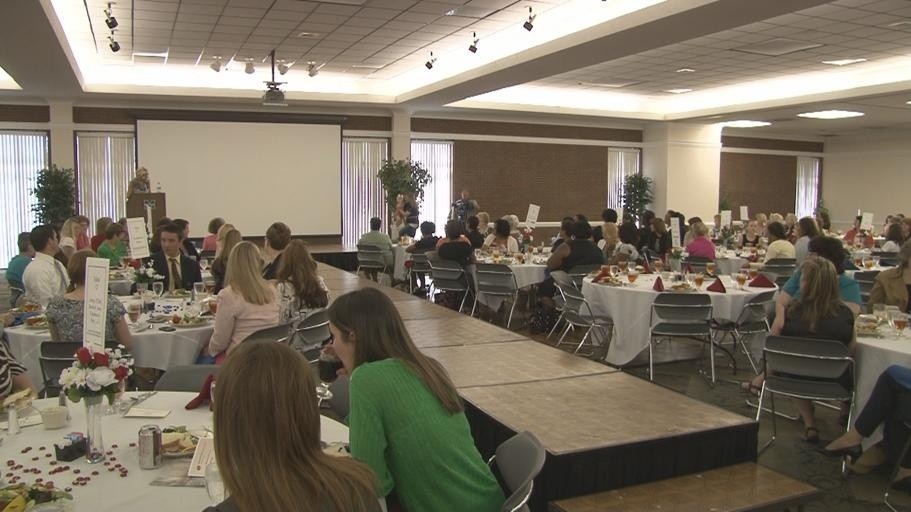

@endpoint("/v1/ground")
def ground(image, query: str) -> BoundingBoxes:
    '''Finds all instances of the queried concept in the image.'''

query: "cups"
[475,241,545,266]
[203,462,228,507]
[200,259,208,271]
[853,255,881,269]
[40,406,69,430]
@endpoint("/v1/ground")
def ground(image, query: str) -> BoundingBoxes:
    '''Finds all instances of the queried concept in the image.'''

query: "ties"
[55,262,67,288]
[172,259,181,289]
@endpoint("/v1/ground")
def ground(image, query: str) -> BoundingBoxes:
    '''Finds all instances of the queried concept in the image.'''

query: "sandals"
[741,382,761,397]
[803,427,818,441]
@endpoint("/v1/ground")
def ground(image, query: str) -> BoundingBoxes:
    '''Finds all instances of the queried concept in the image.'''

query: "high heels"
[815,444,862,464]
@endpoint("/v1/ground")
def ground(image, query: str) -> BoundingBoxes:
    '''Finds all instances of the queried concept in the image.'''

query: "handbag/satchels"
[529,309,566,334]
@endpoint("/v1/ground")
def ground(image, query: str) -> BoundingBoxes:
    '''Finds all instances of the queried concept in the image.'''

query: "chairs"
[0,237,911,512]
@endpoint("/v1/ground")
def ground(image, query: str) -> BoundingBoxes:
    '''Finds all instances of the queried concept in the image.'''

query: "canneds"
[138,425,163,470]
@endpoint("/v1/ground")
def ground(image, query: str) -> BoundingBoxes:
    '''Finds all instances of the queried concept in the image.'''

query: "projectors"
[260,91,287,107]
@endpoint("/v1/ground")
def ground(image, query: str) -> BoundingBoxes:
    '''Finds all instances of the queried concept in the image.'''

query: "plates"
[171,320,207,329]
[24,324,49,331]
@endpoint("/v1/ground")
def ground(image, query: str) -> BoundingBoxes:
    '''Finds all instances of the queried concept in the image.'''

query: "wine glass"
[152,281,164,303]
[607,257,759,291]
[885,307,910,337]
[126,302,140,328]
[194,281,205,302]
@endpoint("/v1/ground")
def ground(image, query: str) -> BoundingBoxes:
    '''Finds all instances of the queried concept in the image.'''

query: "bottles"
[7,401,22,436]
[143,290,156,315]
[156,181,162,193]
[57,390,73,419]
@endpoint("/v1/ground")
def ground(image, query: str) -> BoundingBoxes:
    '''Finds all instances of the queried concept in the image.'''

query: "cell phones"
[160,326,175,331]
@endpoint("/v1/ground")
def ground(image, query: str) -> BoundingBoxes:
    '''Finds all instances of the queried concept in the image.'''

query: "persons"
[127,167,150,198]
[5,215,331,364]
[326,287,507,512]
[202,339,383,512]
[358,191,911,492]
[0,327,32,397]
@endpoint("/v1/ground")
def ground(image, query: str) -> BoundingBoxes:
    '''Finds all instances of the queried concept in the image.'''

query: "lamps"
[468,32,479,53]
[104,2,120,51]
[210,56,318,77]
[425,51,438,69]
[523,5,536,31]
